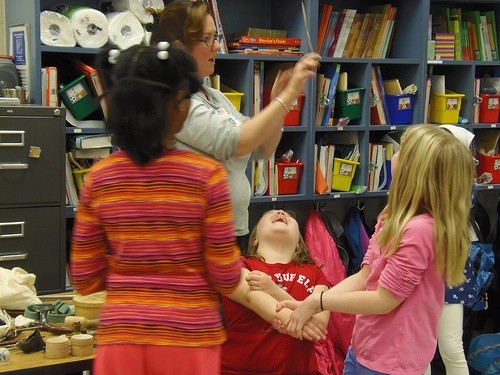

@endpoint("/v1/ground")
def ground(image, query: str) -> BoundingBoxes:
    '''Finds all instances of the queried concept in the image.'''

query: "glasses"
[187,35,221,47]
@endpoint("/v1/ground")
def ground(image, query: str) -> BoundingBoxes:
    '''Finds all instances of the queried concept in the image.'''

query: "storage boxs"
[430,89,466,124]
[71,167,90,191]
[477,150,500,185]
[56,72,98,120]
[331,156,360,191]
[479,93,500,123]
[385,92,417,125]
[275,159,303,194]
[283,93,305,125]
[335,84,366,120]
[220,82,244,113]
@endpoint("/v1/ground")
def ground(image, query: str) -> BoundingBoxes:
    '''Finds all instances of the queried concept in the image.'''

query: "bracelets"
[274,97,289,112]
[321,291,325,311]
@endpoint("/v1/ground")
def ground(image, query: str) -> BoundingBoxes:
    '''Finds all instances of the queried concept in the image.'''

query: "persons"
[149,0,321,249]
[272,123,473,375]
[220,209,331,375]
[70,43,242,375]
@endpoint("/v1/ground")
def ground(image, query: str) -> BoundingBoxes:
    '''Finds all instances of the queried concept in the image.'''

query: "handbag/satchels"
[304,206,350,285]
[443,241,495,311]
[344,205,373,272]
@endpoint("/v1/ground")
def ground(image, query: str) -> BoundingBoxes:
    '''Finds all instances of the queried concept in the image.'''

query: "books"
[41,66,58,107]
[368,142,393,191]
[317,4,397,58]
[266,155,275,196]
[428,7,500,61]
[226,28,304,56]
[77,62,108,118]
[315,64,341,126]
[70,134,112,159]
[370,65,391,125]
[315,143,360,193]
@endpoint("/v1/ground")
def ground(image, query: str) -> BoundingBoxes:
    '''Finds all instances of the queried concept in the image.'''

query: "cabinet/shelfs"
[0,104,67,296]
[5,0,500,204]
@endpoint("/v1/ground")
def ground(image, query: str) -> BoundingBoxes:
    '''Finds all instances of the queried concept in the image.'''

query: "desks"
[0,323,95,375]
